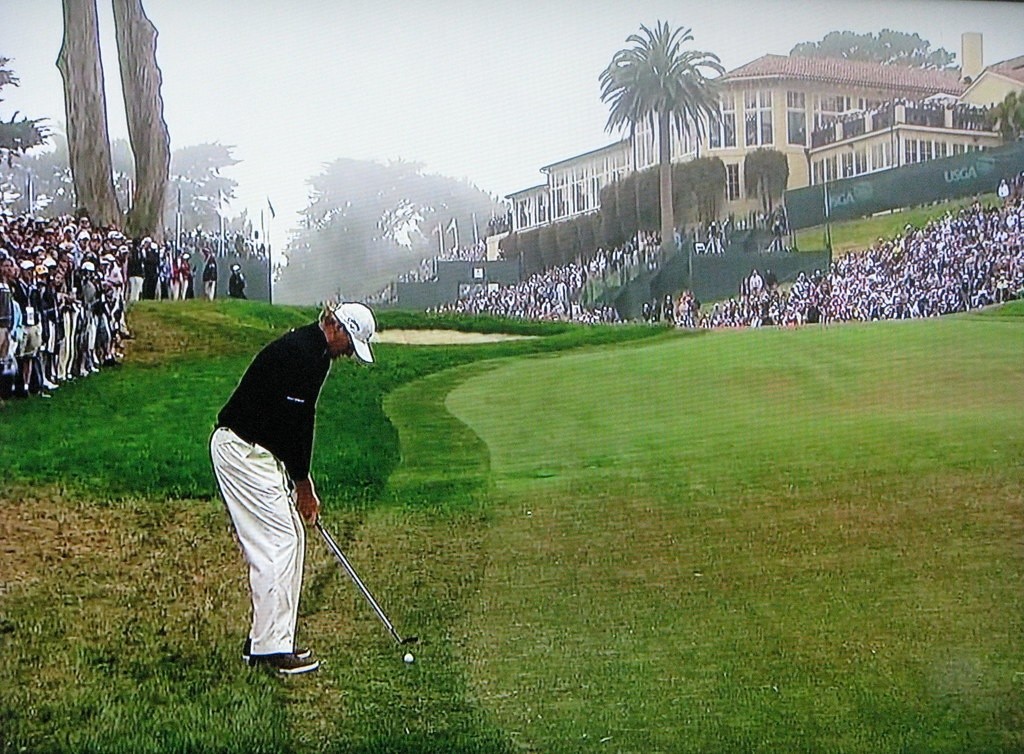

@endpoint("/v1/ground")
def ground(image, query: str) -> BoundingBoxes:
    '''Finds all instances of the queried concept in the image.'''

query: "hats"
[0,214,153,276]
[332,302,376,363]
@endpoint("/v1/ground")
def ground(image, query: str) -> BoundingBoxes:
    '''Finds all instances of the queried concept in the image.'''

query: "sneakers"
[242,637,310,661]
[249,653,319,673]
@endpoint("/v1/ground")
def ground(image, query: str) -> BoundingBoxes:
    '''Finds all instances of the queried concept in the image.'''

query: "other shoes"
[36,350,125,398]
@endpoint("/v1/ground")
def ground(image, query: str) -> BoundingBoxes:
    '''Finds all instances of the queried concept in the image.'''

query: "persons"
[811,97,999,147]
[641,171,1024,328]
[426,203,798,324]
[0,210,265,400]
[209,302,375,676]
